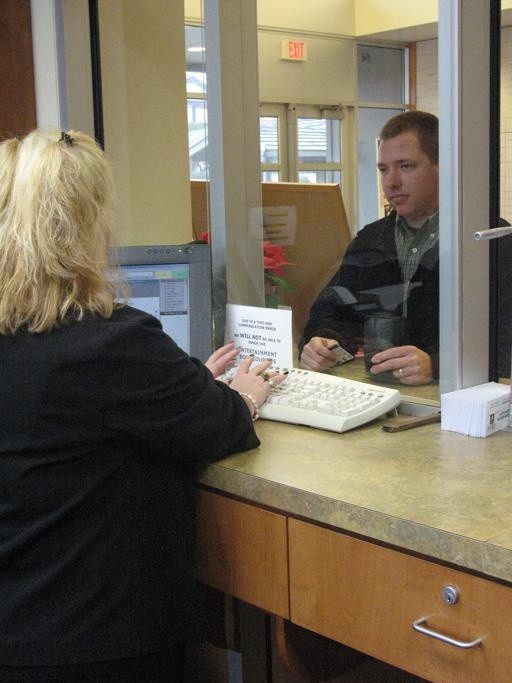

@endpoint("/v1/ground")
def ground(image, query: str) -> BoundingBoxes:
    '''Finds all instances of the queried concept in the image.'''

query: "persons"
[0,127,289,682]
[297,112,511,386]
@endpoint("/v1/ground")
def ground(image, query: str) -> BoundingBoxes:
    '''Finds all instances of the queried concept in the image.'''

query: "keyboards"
[214,363,402,434]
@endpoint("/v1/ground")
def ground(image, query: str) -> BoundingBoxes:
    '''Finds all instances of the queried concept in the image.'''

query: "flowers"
[187,224,297,310]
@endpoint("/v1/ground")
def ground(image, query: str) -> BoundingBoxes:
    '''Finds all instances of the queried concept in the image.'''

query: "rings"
[267,378,276,389]
[259,371,269,382]
[399,368,406,377]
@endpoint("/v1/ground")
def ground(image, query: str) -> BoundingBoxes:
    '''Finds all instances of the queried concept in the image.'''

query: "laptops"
[101,244,212,366]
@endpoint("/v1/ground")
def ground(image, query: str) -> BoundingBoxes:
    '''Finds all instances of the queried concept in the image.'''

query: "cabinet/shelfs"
[188,487,290,623]
[288,514,511,682]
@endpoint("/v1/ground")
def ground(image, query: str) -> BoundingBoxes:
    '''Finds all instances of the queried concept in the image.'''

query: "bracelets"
[239,391,259,422]
